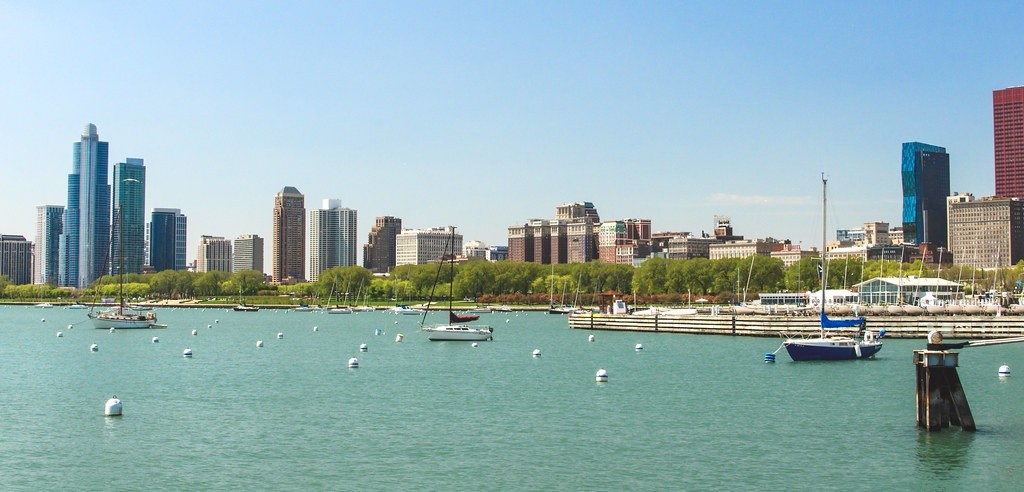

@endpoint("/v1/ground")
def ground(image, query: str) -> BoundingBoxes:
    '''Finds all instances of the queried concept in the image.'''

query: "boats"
[396,307,421,315]
[35,302,54,309]
[490,305,513,312]
[68,304,89,310]
[449,311,480,324]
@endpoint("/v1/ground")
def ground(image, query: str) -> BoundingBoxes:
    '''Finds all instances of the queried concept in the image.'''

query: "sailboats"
[420,225,493,341]
[726,254,1023,317]
[233,284,260,312]
[783,170,883,362]
[550,263,601,315]
[88,205,157,329]
[296,274,376,314]
